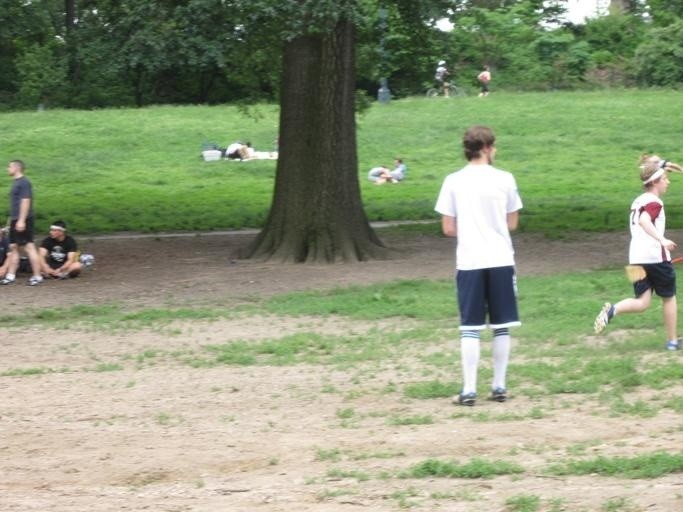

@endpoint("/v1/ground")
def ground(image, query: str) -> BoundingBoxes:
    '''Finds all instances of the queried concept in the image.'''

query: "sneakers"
[484,386,508,402]
[666,340,683,351]
[24,276,44,286]
[0,278,16,285]
[594,302,616,334]
[451,390,477,406]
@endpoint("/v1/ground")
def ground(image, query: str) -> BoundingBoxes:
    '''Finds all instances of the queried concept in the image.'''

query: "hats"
[640,163,665,185]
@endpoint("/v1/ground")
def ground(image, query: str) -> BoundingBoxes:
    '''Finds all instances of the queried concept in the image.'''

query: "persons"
[432,59,450,99]
[367,164,390,185]
[640,152,683,173]
[592,158,683,353]
[38,220,81,281]
[431,125,524,406]
[0,231,12,277]
[0,159,43,287]
[477,63,490,97]
[380,158,405,184]
[224,141,250,159]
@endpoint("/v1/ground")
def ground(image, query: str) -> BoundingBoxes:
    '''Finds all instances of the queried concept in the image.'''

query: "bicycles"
[425,82,457,98]
